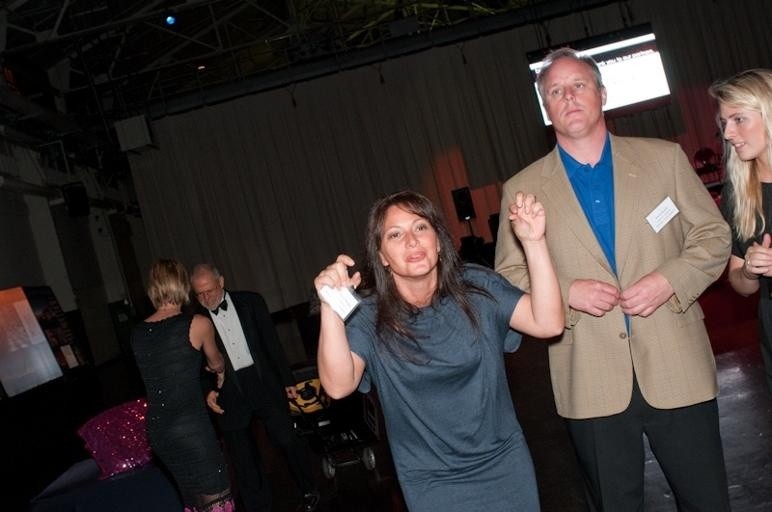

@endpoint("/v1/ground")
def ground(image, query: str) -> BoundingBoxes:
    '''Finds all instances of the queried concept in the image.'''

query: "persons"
[189,265,324,511]
[132,260,236,511]
[708,67,772,390]
[493,47,731,512]
[313,190,564,511]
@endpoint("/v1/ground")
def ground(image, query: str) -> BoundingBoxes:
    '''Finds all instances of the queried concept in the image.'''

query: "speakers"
[450,187,477,223]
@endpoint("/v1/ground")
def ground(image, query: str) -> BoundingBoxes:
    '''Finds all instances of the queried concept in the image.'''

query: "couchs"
[25,435,195,512]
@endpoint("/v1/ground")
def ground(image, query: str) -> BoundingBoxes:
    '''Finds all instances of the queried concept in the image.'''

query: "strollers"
[284,362,381,480]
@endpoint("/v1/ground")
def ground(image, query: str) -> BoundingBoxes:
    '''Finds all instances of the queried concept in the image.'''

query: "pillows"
[79,396,156,479]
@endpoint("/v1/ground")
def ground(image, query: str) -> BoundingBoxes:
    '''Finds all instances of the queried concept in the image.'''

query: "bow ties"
[210,288,229,315]
